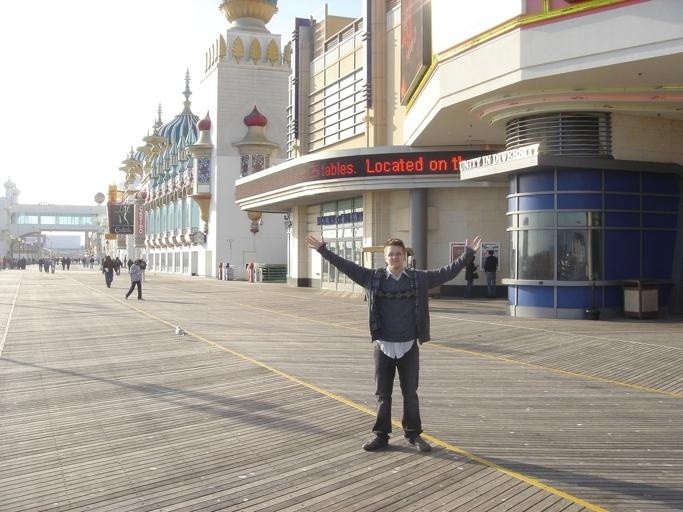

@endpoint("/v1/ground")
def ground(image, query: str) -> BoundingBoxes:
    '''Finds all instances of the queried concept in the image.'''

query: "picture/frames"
[480,242,500,272]
[449,242,466,272]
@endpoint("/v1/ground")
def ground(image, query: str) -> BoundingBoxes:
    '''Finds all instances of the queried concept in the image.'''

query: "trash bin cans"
[622,280,664,319]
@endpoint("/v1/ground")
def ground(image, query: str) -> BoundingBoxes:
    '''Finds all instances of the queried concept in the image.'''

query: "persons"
[2,254,148,301]
[465,256,479,298]
[304,234,483,453]
[485,249,498,299]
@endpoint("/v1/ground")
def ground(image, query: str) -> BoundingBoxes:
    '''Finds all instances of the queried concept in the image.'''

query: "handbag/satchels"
[472,273,477,279]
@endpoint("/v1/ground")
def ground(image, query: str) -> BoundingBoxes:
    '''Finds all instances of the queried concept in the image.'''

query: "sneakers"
[363,435,388,451]
[407,436,431,452]
